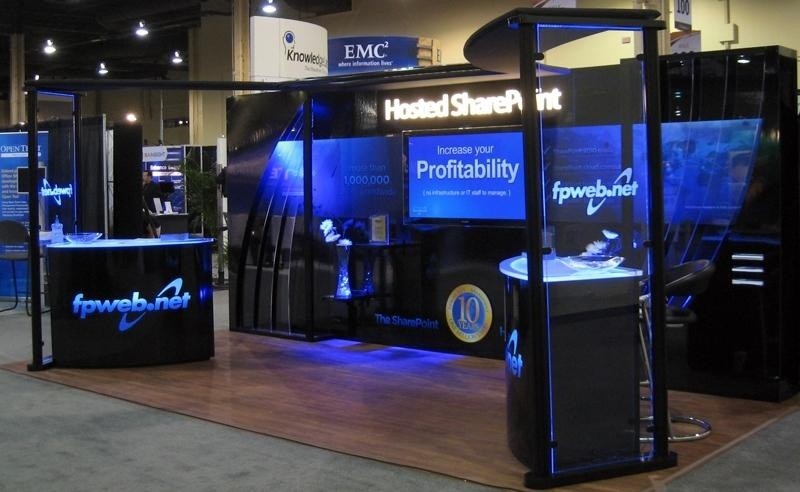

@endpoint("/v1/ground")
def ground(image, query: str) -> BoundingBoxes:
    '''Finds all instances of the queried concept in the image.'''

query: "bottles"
[51,216,63,243]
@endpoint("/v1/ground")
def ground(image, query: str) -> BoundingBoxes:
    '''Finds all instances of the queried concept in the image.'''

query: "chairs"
[0,219,52,318]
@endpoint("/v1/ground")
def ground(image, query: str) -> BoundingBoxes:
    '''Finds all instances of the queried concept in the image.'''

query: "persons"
[142,170,162,210]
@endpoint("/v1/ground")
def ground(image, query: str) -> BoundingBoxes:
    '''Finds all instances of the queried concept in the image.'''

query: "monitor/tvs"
[542,123,625,231]
[17,167,47,194]
[630,116,782,248]
[400,124,526,231]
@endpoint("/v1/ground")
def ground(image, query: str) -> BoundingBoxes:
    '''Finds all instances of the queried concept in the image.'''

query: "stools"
[635,258,716,442]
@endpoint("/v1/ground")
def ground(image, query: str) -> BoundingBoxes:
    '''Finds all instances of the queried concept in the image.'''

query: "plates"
[63,233,102,242]
[558,255,625,274]
